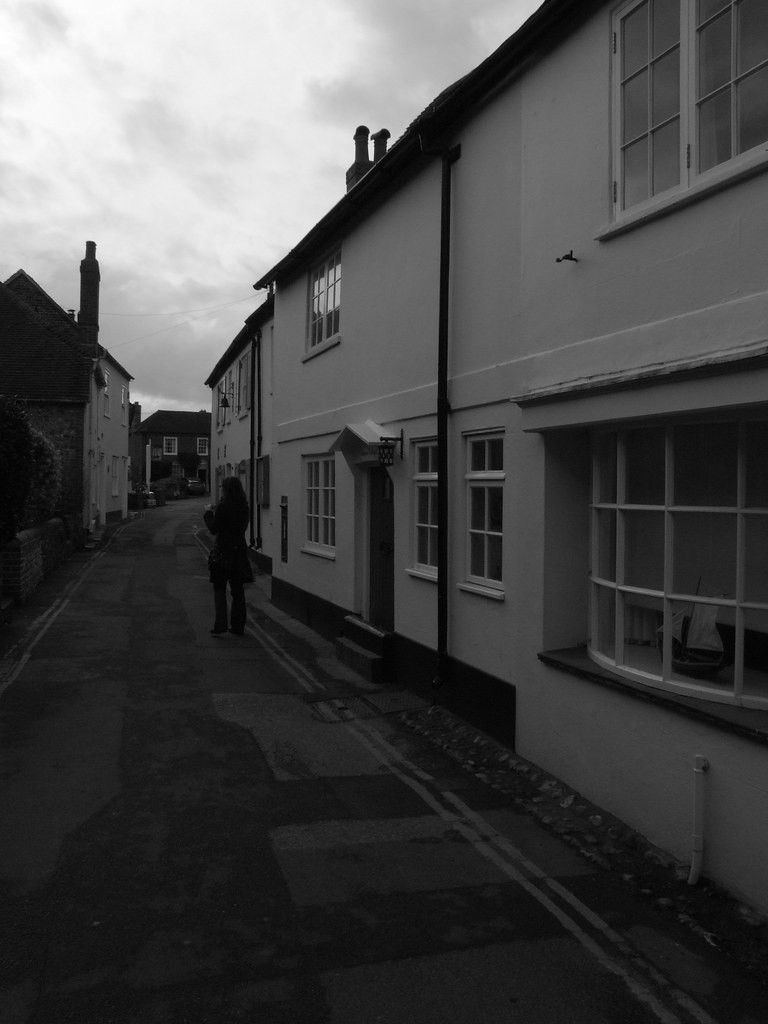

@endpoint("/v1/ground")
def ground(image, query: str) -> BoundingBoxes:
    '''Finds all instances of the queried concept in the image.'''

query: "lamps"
[376,428,403,466]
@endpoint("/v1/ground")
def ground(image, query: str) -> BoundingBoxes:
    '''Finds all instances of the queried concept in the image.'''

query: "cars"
[173,477,206,496]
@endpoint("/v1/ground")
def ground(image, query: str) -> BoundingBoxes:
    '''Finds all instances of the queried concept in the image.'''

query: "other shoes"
[210,629,227,634]
[229,627,245,634]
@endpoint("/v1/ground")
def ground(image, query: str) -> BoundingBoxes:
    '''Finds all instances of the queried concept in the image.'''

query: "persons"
[204,475,255,637]
